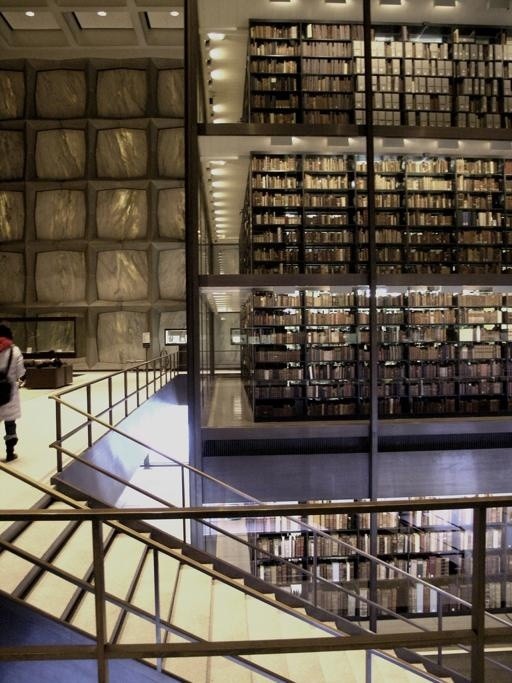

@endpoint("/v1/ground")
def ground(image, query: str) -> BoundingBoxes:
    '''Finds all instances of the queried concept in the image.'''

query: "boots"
[4,434,18,462]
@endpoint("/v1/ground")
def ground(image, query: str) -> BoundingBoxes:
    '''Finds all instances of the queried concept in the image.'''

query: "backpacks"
[0,371,12,406]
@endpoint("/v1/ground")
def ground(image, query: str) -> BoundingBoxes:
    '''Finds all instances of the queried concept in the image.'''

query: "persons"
[0,323,28,461]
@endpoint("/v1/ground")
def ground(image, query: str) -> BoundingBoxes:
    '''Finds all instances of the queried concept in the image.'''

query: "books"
[244,25,512,130]
[248,154,512,274]
[240,292,512,417]
[255,506,512,617]
